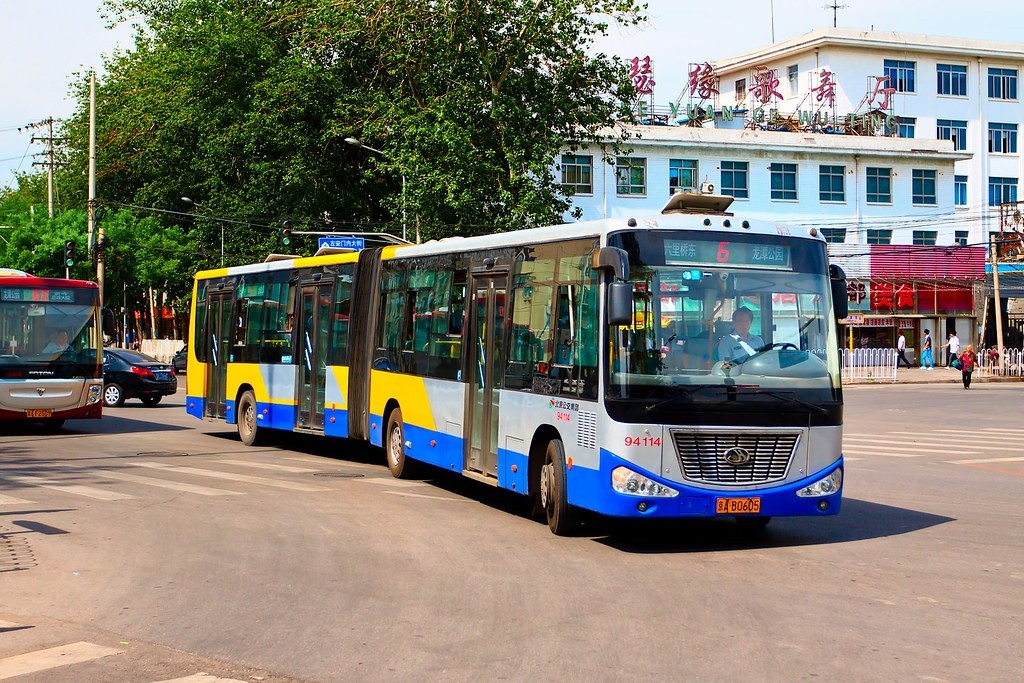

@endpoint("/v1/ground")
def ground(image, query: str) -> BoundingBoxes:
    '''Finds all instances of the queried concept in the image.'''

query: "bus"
[185,191,849,535]
[0,268,116,433]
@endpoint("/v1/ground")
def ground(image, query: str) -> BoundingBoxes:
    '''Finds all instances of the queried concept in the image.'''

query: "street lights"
[344,138,406,240]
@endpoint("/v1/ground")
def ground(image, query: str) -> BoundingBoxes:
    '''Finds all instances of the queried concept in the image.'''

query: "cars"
[171,344,188,374]
[81,347,177,408]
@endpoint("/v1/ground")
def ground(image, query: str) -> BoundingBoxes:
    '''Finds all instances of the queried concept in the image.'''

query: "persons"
[42,329,73,353]
[959,345,979,390]
[718,306,764,363]
[846,333,860,348]
[940,330,961,369]
[861,333,869,348]
[920,329,934,370]
[132,338,138,350]
[897,329,911,369]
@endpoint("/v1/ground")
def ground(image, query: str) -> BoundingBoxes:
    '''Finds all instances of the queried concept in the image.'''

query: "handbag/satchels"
[951,359,962,371]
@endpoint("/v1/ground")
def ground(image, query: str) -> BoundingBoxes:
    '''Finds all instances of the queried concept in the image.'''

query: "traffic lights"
[65,239,76,268]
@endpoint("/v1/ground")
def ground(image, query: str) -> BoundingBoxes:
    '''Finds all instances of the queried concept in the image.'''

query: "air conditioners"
[701,182,714,193]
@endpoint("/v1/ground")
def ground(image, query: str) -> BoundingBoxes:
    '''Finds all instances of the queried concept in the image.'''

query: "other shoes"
[920,366,926,370]
[927,367,933,370]
